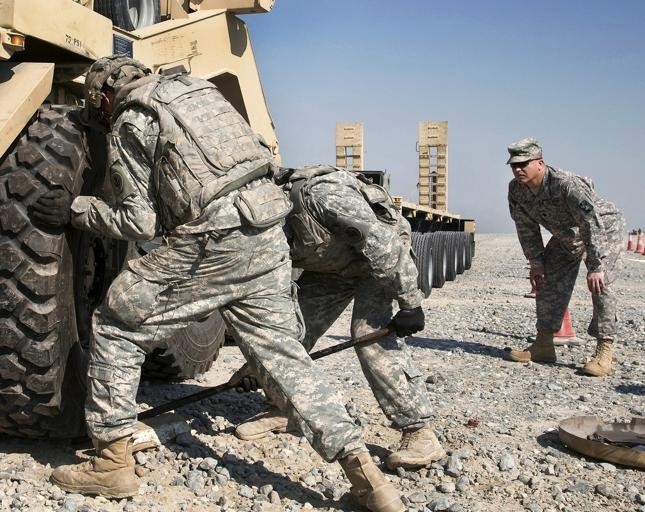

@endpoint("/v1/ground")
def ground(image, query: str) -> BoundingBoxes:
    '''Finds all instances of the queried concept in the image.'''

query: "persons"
[30,53,409,512]
[225,132,449,467]
[500,136,628,380]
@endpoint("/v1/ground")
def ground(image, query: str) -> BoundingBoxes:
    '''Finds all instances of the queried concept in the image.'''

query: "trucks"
[0,3,481,442]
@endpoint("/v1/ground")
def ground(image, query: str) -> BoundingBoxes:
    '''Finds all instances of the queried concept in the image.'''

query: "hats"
[506,137,542,165]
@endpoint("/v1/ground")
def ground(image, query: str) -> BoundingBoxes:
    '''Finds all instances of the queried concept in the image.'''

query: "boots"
[504,333,558,362]
[584,340,615,375]
[340,453,407,512]
[47,440,141,500]
[388,426,446,468]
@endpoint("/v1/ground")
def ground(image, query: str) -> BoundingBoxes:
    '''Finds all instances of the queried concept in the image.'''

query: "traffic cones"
[530,295,585,347]
[641,246,645,256]
[634,232,643,255]
[625,231,633,253]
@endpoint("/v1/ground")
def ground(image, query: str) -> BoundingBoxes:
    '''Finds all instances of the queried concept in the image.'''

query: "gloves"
[390,307,425,337]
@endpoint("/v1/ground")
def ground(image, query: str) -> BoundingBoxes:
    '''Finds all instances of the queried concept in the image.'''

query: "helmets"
[84,54,147,110]
